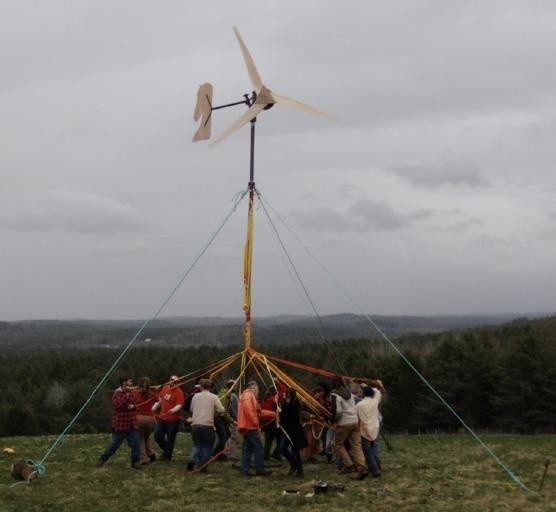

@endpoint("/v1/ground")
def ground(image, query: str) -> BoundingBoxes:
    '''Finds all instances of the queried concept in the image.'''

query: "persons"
[95,375,388,480]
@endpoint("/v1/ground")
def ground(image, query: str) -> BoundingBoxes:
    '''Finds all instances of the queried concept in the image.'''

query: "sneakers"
[131,463,144,470]
[356,469,369,481]
[338,465,358,475]
[97,458,105,468]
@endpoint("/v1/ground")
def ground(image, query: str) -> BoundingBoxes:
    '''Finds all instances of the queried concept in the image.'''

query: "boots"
[283,449,304,478]
